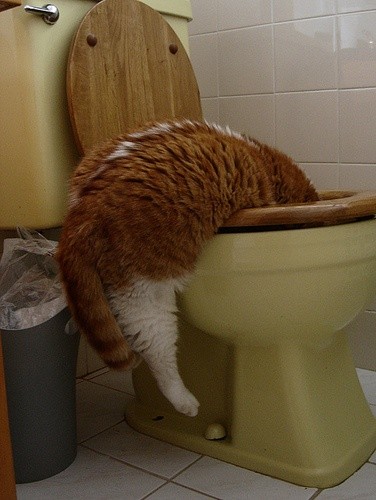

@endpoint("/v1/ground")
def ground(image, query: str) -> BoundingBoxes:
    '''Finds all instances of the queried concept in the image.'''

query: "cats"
[56,118,320,418]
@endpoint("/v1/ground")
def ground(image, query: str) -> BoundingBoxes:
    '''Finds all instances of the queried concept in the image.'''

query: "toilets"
[2,2,375,489]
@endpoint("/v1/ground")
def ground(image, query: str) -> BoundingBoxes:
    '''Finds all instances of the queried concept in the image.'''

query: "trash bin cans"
[4,223,78,484]
